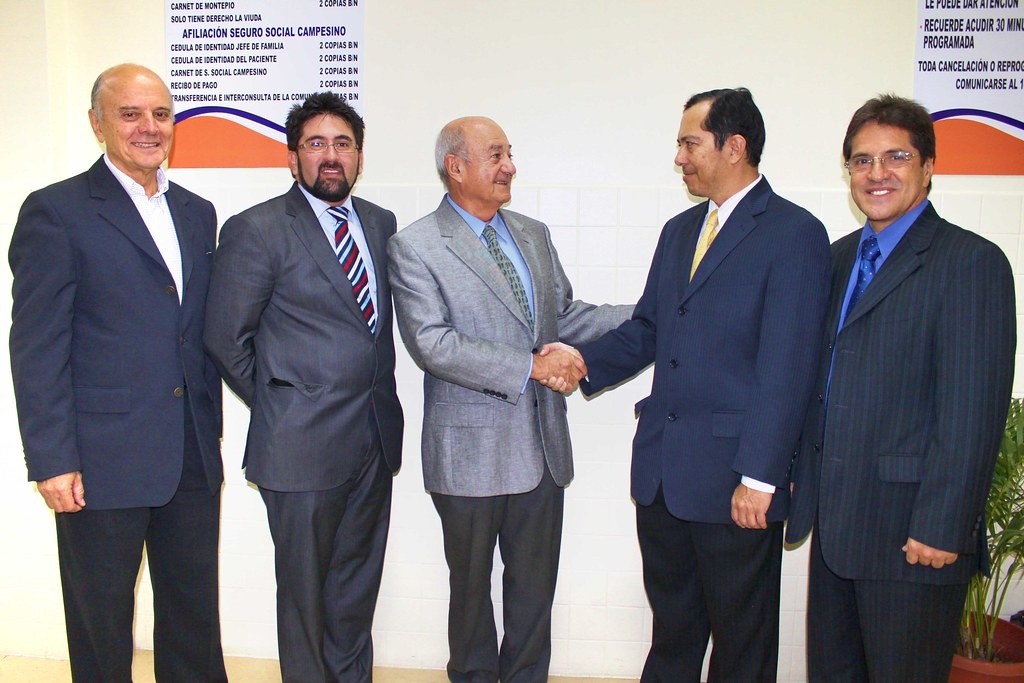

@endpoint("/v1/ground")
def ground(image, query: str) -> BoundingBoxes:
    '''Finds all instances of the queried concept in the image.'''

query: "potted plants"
[946,396,1024,683]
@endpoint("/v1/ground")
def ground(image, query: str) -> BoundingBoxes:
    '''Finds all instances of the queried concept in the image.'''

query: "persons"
[5,61,230,683]
[201,91,405,683]
[785,90,1018,683]
[386,114,639,683]
[538,87,831,683]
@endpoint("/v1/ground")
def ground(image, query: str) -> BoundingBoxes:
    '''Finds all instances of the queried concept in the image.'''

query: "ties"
[840,237,881,328]
[690,208,719,283]
[326,207,376,336]
[482,226,534,333]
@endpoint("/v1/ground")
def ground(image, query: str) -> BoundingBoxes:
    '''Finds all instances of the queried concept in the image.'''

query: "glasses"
[296,141,359,153]
[843,151,921,170]
